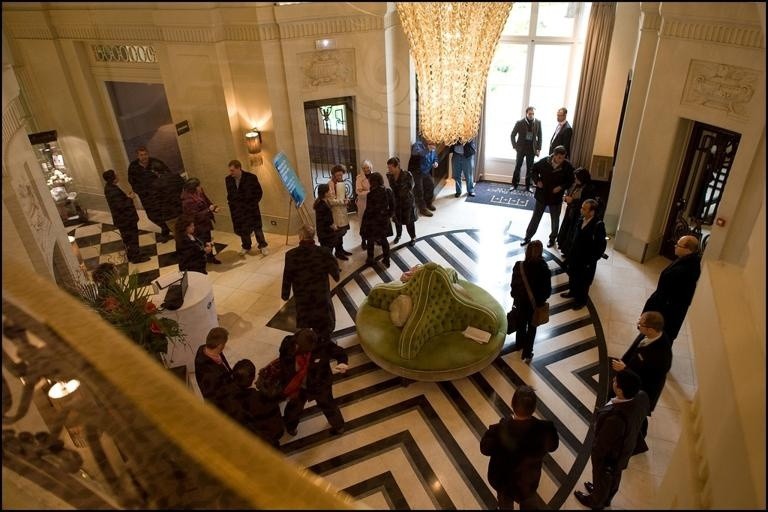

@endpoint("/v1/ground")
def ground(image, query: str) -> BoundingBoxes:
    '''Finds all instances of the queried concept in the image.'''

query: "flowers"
[65,245,192,369]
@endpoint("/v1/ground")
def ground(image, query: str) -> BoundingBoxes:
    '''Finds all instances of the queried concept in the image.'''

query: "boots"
[334,242,352,260]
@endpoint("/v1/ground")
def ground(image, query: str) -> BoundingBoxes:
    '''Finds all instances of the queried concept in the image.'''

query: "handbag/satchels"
[532,303,549,325]
[256,358,288,400]
[387,206,396,218]
[507,310,518,334]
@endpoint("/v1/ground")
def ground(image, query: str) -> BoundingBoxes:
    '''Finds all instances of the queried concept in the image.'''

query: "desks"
[135,270,219,370]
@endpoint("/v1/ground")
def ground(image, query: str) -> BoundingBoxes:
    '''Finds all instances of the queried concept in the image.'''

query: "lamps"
[396,1,513,147]
[245,126,262,155]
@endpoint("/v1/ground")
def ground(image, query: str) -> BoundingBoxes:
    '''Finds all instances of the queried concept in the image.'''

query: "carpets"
[464,180,561,215]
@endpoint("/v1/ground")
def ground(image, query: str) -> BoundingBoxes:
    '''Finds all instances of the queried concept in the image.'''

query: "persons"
[611,311,673,418]
[510,239,551,365]
[408,134,439,217]
[195,326,349,447]
[517,145,606,310]
[312,157,417,274]
[620,235,702,364]
[102,145,222,275]
[92,264,132,307]
[549,107,573,160]
[479,385,559,511]
[573,367,651,512]
[280,223,341,338]
[509,106,542,193]
[445,138,476,198]
[224,159,269,257]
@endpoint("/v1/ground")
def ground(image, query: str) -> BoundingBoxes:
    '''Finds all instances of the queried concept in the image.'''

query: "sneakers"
[240,248,248,255]
[261,247,268,256]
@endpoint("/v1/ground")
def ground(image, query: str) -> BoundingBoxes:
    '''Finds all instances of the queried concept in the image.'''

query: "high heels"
[521,350,533,360]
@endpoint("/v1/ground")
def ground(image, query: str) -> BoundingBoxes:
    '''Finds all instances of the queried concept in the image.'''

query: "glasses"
[638,322,652,328]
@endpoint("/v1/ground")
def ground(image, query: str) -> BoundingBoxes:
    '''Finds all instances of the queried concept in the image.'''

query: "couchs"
[357,263,508,384]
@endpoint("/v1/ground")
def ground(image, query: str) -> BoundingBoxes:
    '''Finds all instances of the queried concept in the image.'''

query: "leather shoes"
[527,188,534,192]
[509,186,515,191]
[520,239,530,246]
[286,426,298,436]
[394,236,401,243]
[335,422,344,434]
[420,204,436,216]
[547,240,555,247]
[411,238,416,246]
[455,193,461,197]
[139,256,150,261]
[207,257,221,264]
[361,241,367,250]
[561,293,588,310]
[382,259,390,267]
[574,482,610,510]
[161,234,170,242]
[376,239,382,245]
[469,192,475,196]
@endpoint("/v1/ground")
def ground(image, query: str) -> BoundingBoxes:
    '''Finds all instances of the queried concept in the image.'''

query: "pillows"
[452,282,473,302]
[390,293,414,327]
[372,277,402,288]
